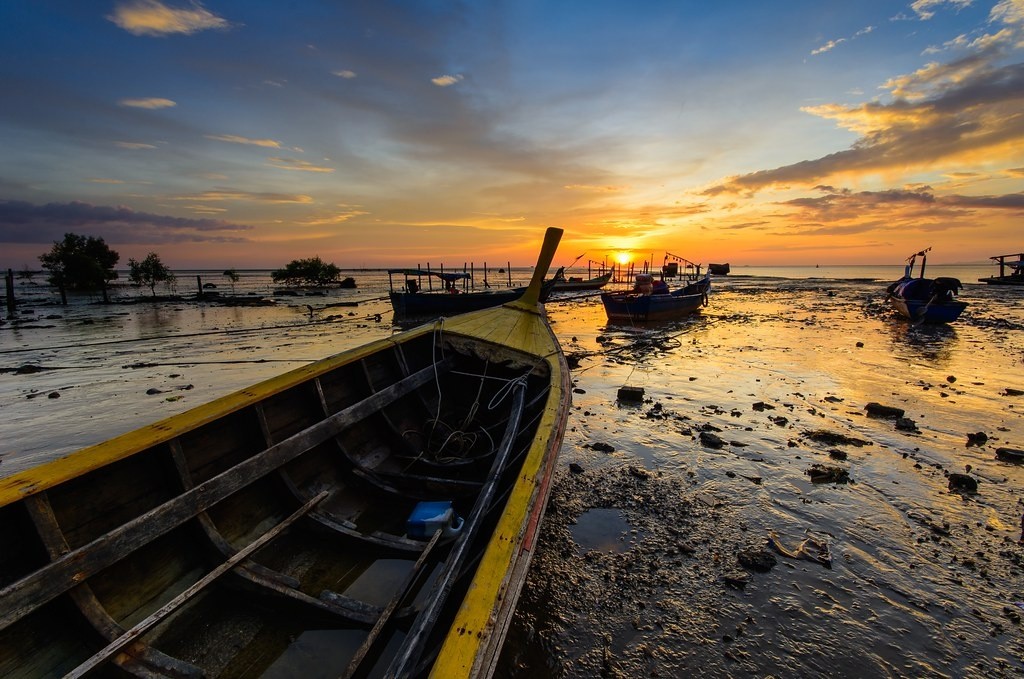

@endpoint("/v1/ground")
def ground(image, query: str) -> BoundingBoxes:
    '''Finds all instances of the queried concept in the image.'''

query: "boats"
[977,253,1024,286]
[543,268,613,289]
[0,228,572,679]
[601,253,713,319]
[887,247,967,320]
[385,262,529,312]
